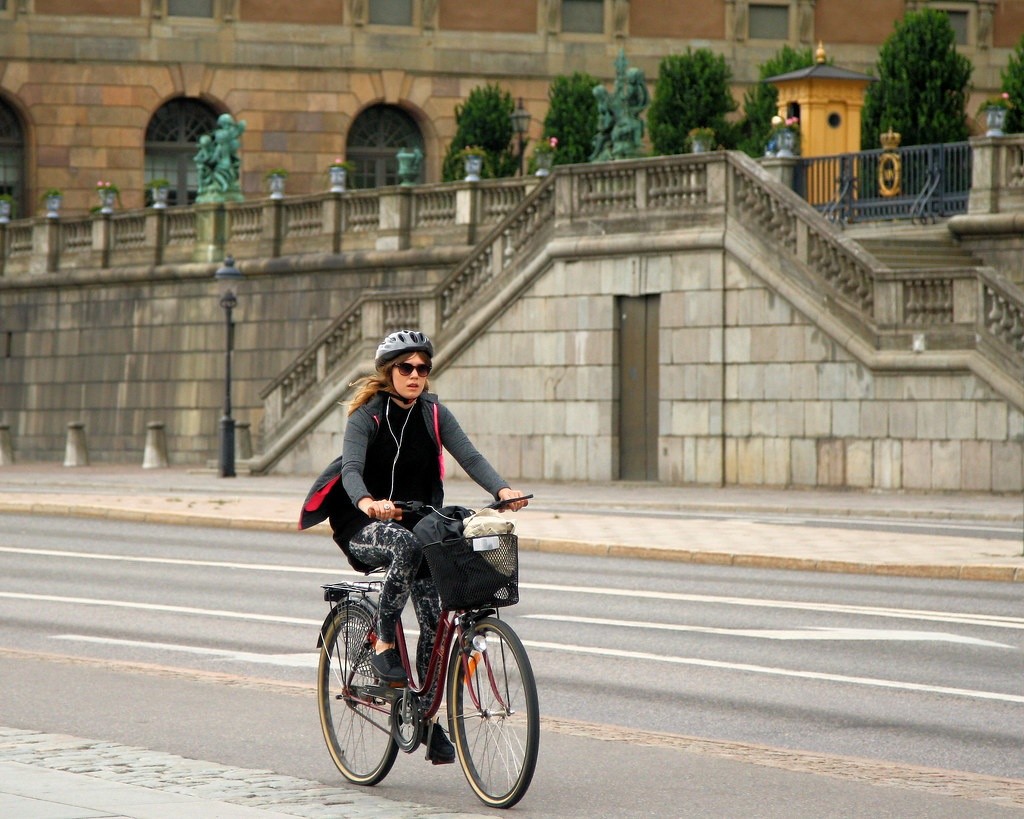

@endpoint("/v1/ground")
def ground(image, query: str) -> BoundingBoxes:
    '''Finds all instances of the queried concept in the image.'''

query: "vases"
[776,133,797,157]
[535,153,554,177]
[98,189,115,214]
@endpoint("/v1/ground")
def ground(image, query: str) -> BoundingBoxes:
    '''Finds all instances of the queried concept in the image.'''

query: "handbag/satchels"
[413,505,519,610]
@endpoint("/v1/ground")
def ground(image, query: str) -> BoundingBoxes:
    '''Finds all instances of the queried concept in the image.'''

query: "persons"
[590,64,648,156]
[194,113,248,192]
[298,329,527,764]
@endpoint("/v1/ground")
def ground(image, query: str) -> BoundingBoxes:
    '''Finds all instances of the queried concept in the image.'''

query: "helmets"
[374,329,433,367]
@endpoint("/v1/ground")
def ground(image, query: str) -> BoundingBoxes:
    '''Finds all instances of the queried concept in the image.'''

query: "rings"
[384,505,390,509]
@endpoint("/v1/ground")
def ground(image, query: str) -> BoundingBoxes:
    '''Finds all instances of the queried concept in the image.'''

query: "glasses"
[393,363,430,378]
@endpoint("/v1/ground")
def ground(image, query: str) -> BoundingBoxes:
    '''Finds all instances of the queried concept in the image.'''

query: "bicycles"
[314,493,542,810]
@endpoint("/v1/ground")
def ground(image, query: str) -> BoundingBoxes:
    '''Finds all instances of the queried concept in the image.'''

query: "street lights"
[212,254,243,480]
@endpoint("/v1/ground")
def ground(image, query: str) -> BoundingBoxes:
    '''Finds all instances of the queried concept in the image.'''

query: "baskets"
[422,534,519,611]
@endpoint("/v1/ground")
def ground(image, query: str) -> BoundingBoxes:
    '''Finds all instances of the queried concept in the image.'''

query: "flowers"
[535,137,560,150]
[95,180,116,190]
[977,93,1014,109]
[770,115,800,132]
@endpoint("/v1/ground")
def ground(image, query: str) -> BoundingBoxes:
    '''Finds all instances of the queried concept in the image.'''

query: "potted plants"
[41,188,63,218]
[458,145,485,182]
[985,110,1006,137]
[0,194,17,223]
[263,168,288,200]
[324,159,356,194]
[686,128,715,153]
[395,145,422,187]
[144,179,171,209]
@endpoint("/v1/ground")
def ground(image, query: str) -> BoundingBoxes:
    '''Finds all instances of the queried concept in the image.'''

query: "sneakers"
[369,649,407,683]
[414,716,455,760]
